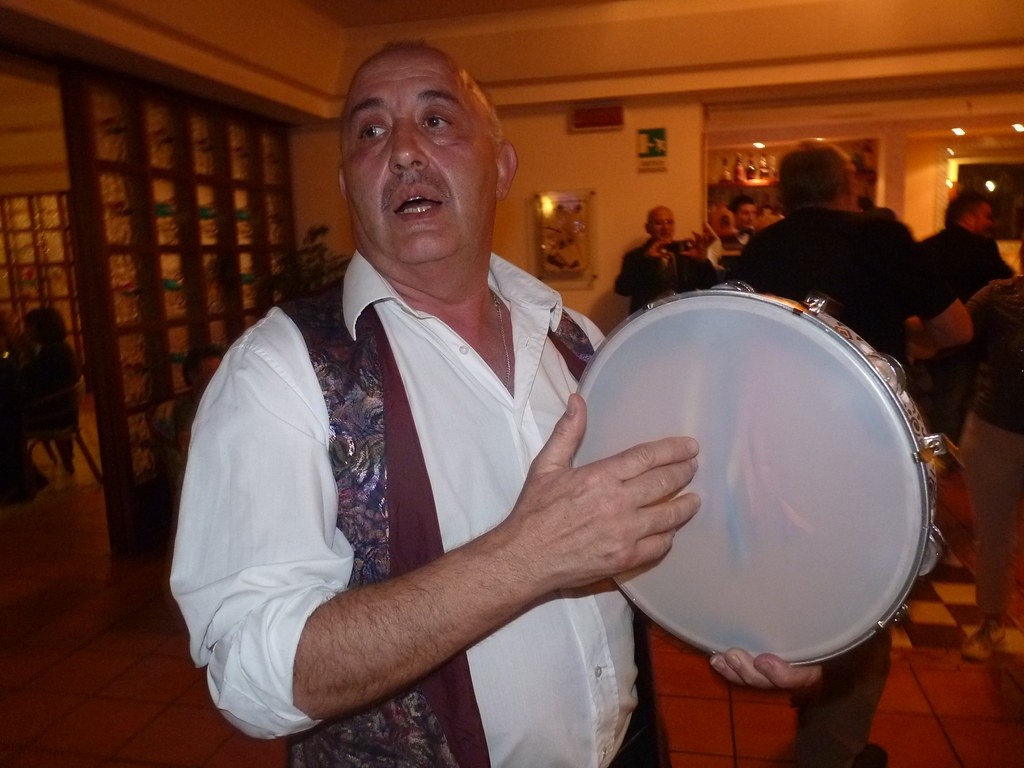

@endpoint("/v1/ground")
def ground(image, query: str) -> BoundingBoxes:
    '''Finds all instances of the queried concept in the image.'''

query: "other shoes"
[961,621,1006,659]
[11,469,48,502]
[852,742,889,768]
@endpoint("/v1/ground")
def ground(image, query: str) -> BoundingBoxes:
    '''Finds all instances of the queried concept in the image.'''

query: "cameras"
[666,240,691,253]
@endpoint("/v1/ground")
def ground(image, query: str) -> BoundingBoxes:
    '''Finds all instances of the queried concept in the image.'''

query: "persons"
[0,308,81,504]
[168,39,822,768]
[614,137,1024,768]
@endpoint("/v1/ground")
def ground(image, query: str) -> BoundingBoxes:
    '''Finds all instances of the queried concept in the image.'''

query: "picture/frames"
[525,189,600,292]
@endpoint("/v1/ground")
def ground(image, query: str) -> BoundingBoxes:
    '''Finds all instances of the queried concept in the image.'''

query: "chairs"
[145,395,186,558]
[20,373,102,484]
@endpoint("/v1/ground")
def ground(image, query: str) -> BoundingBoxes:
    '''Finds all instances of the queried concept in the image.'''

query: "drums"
[572,287,938,667]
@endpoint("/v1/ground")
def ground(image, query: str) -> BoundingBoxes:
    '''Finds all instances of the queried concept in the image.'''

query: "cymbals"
[569,280,951,671]
